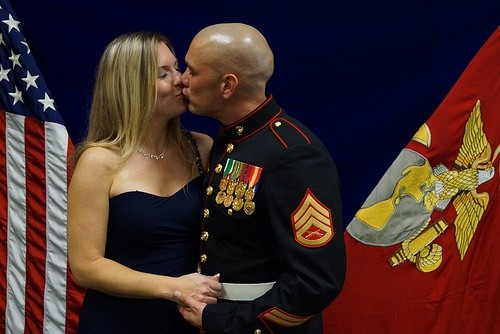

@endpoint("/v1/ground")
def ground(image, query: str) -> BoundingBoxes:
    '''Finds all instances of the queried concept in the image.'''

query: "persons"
[66,31,221,334]
[176,22,346,334]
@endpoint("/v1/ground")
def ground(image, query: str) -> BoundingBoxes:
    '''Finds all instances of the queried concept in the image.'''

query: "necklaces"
[136,142,169,160]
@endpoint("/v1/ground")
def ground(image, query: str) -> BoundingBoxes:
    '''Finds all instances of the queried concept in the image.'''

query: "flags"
[0,0,86,334]
[323,24,500,334]
